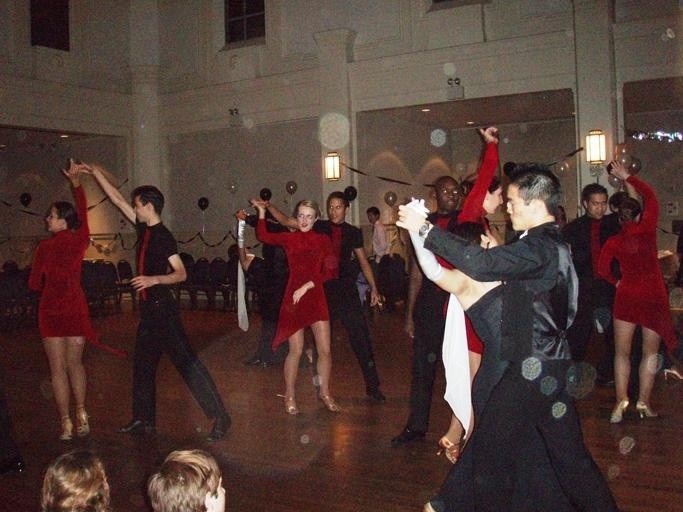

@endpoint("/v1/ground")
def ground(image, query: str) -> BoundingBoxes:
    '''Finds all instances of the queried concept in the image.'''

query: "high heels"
[59,417,73,440]
[610,398,629,424]
[664,367,682,380]
[636,400,657,418]
[76,411,90,436]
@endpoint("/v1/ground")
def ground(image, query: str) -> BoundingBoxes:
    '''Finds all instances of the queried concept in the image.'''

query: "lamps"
[585,128,606,163]
[325,150,341,182]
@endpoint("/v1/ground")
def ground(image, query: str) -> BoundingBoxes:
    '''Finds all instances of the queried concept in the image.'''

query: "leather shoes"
[246,353,462,464]
[118,419,154,432]
[207,416,231,441]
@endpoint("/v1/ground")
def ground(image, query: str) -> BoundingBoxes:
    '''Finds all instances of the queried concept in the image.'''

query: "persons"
[24,157,92,441]
[144,448,227,512]
[38,448,112,512]
[77,160,233,444]
[391,124,683,511]
[235,191,391,413]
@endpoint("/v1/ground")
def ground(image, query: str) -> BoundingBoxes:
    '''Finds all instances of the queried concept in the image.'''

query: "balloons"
[384,191,396,207]
[286,181,296,194]
[260,187,271,200]
[344,186,357,201]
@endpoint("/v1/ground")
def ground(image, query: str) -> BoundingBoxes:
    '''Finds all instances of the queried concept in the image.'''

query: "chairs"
[117,259,137,301]
[210,256,230,309]
[194,257,216,308]
[79,261,104,311]
[23,263,42,324]
[0,260,32,326]
[175,252,197,310]
[94,260,122,311]
[246,257,265,305]
[225,254,238,309]
[377,254,409,307]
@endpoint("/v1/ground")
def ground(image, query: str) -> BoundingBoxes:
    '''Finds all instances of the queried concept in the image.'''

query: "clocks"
[429,127,448,148]
